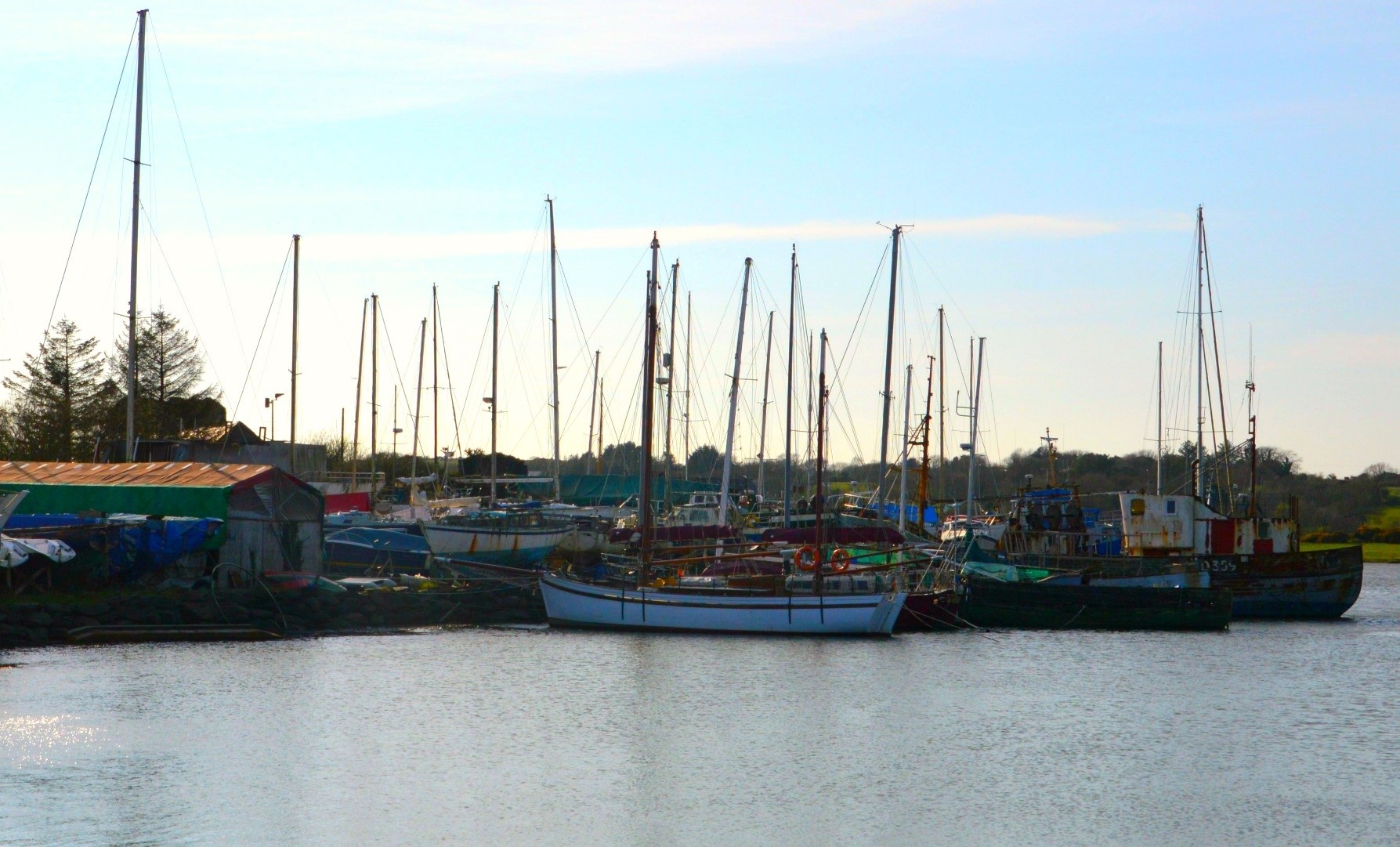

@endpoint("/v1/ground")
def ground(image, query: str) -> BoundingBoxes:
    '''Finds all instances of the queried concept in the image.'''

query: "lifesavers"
[831,548,850,572]
[795,545,820,571]
[746,514,759,527]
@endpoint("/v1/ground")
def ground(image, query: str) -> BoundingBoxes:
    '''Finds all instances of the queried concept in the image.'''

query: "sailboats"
[0,6,1363,637]
